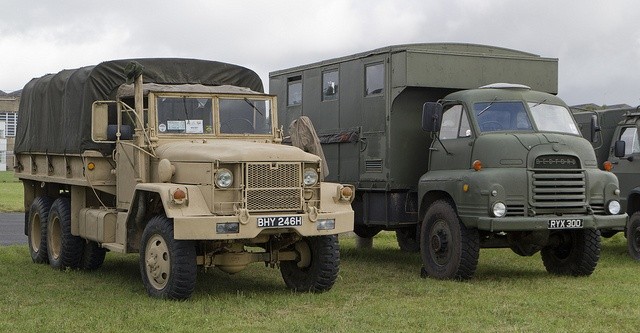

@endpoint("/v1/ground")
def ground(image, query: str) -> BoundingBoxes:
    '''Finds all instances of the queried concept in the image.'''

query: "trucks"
[568,103,640,257]
[269,42,628,281]
[13,58,355,299]
